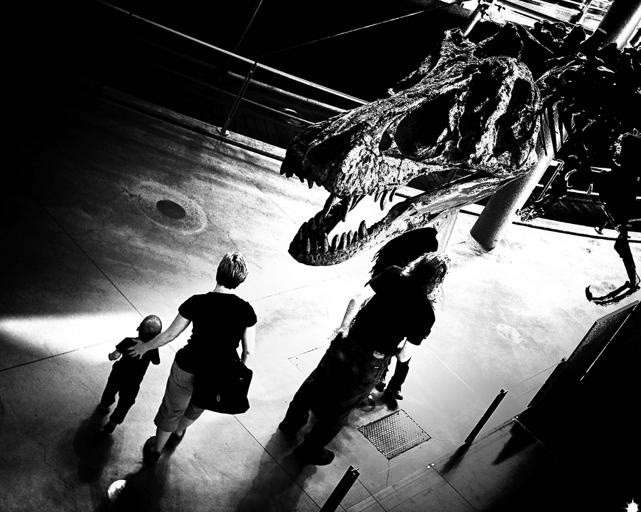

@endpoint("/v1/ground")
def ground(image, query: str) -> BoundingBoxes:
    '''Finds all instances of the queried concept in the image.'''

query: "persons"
[95,314,161,435]
[277,250,449,466]
[128,251,258,467]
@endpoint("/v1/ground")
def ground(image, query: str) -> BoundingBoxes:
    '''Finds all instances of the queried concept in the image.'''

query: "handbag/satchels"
[193,357,253,415]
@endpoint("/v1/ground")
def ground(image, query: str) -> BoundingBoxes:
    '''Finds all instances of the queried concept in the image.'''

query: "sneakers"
[143,427,185,465]
[278,411,336,465]
[93,403,124,436]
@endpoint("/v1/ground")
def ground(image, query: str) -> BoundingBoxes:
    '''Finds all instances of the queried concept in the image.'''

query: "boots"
[374,346,414,401]
